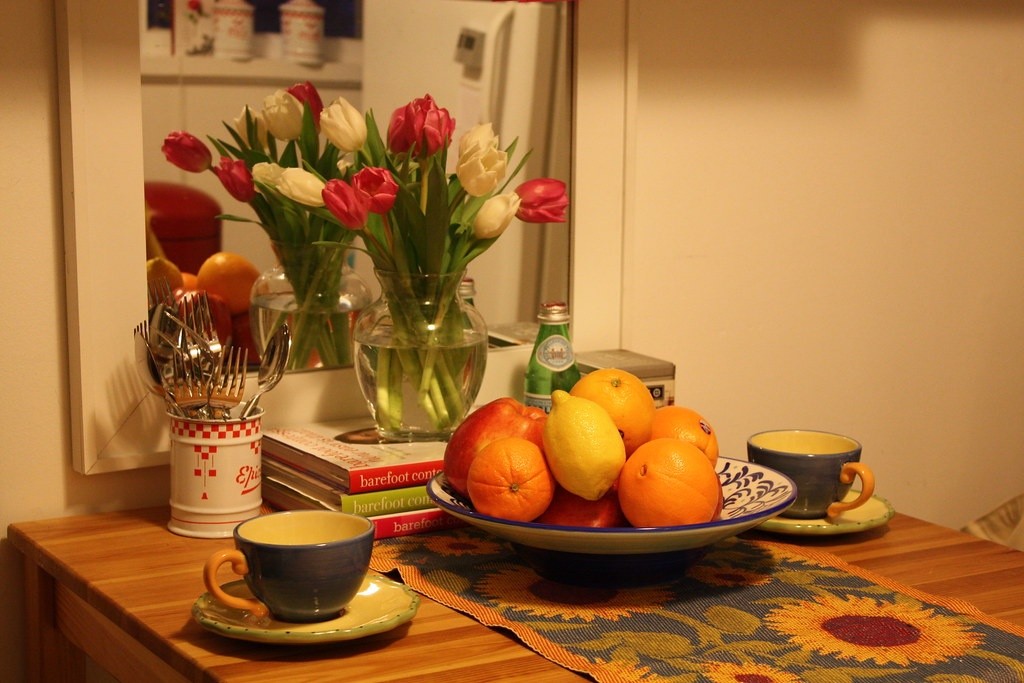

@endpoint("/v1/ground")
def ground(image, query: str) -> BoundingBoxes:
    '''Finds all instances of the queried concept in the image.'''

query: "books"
[261,402,487,540]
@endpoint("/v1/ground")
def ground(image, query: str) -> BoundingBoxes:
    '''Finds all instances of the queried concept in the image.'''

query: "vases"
[352,266,489,442]
[248,239,373,373]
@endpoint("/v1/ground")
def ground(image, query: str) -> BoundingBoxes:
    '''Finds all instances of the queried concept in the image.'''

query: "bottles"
[461,279,478,328]
[525,301,580,412]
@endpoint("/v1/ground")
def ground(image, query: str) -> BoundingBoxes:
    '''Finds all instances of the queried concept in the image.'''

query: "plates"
[192,573,422,646]
[751,486,894,535]
[424,457,798,579]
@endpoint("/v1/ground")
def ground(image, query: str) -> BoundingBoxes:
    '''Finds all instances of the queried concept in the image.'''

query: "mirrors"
[56,0,637,477]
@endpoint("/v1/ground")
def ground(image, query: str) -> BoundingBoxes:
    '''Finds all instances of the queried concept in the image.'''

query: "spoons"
[133,303,293,417]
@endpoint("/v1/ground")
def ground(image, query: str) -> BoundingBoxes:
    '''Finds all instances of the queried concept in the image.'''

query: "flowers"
[274,93,571,433]
[160,81,361,372]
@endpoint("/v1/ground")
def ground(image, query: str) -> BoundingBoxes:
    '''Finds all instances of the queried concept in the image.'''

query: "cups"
[746,428,874,519]
[202,509,376,624]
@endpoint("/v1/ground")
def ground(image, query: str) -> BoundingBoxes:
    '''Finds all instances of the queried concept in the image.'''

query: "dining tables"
[7,499,1024,683]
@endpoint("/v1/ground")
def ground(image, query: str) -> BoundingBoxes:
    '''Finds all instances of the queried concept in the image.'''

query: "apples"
[441,392,559,499]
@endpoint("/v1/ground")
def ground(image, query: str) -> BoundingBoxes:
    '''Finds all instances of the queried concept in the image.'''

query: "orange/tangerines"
[465,369,721,529]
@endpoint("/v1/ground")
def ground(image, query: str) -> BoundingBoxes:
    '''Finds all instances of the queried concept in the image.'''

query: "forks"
[146,277,178,314]
[137,289,251,421]
